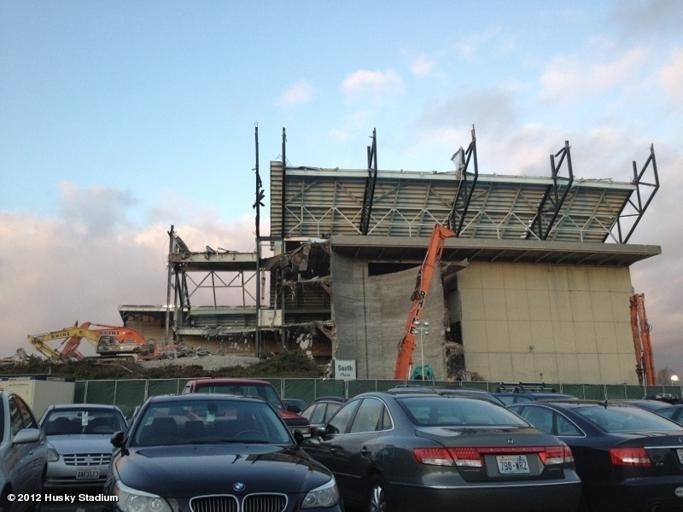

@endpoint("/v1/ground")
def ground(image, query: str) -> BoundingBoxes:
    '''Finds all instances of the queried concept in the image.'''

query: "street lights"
[413,320,430,381]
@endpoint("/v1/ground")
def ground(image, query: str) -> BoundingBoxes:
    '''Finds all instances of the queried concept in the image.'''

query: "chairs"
[527,411,549,432]
[437,414,490,425]
[144,417,203,444]
[49,417,114,433]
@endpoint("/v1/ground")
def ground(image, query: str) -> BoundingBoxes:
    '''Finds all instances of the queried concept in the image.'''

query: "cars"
[0,378,683,512]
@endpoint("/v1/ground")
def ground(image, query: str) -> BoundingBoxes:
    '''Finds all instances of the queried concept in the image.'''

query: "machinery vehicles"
[27,321,159,363]
